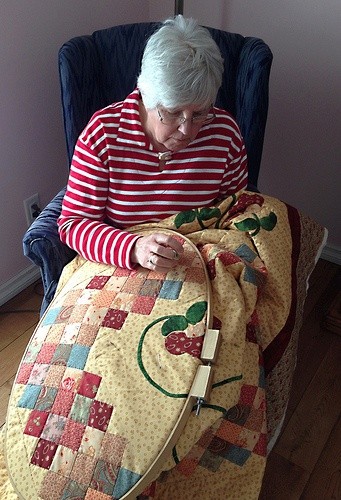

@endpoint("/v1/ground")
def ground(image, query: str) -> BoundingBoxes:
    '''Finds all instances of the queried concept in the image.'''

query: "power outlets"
[23,193,41,225]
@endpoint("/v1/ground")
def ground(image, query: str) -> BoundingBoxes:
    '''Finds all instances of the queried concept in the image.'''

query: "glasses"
[155,104,216,125]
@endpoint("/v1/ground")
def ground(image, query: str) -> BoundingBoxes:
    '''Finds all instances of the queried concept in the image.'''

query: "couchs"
[23,22,273,319]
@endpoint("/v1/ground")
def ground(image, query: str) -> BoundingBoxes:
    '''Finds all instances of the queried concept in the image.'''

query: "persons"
[56,14,248,272]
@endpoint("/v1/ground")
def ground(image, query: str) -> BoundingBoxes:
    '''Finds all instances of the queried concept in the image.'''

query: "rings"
[172,249,179,261]
